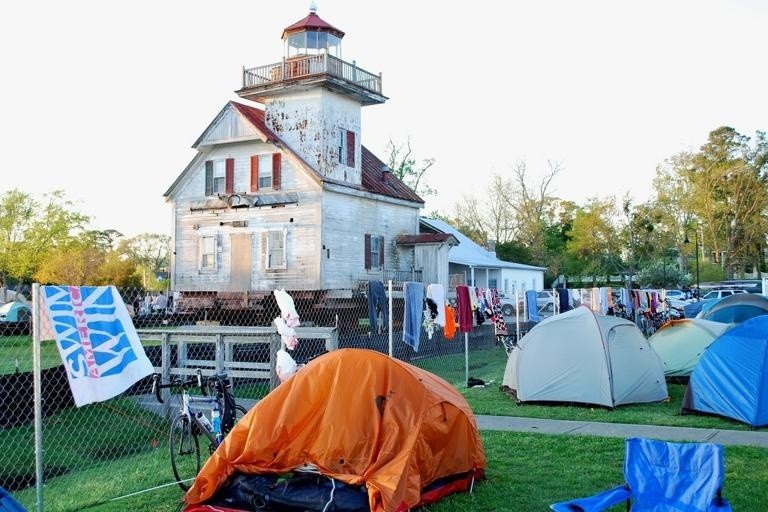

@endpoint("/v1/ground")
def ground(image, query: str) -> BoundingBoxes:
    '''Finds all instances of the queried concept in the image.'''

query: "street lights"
[682,227,700,300]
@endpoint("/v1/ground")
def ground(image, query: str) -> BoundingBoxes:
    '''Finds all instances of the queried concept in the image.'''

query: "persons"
[144,292,152,309]
[152,291,169,313]
[132,295,140,315]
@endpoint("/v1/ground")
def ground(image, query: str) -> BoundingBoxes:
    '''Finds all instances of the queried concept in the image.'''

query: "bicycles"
[150,369,249,494]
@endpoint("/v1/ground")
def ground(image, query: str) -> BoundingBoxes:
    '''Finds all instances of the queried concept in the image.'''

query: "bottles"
[196,411,214,433]
[211,406,220,433]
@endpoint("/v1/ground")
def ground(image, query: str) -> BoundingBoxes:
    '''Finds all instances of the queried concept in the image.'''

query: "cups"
[216,434,224,445]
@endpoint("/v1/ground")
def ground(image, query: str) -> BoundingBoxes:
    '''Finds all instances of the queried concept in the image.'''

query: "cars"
[664,277,763,318]
[495,288,621,317]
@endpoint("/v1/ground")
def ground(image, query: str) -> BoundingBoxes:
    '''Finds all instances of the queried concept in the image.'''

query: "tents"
[682,298,711,318]
[175,348,485,511]
[498,304,670,410]
[680,314,767,429]
[692,293,767,324]
[0,302,33,336]
[647,318,735,385]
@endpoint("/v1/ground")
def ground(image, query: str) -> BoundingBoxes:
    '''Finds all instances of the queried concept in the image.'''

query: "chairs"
[549,437,734,512]
